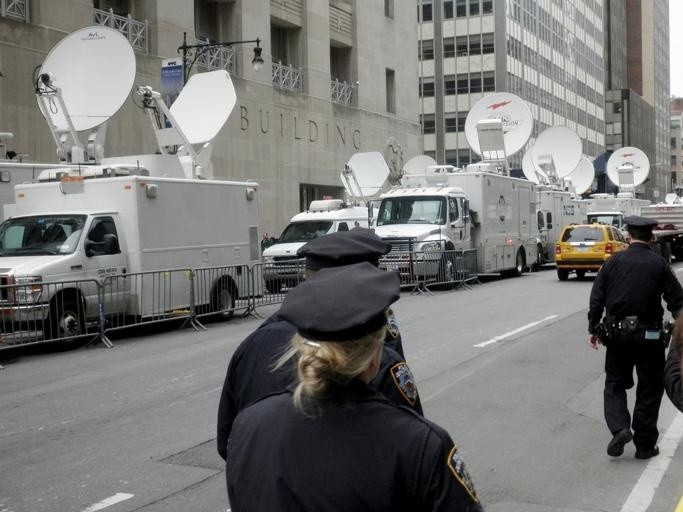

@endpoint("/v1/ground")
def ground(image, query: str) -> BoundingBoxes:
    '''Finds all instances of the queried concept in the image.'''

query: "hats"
[297,226,392,267]
[624,215,659,231]
[275,261,401,343]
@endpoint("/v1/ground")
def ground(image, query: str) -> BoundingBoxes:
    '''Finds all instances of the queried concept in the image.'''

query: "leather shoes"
[635,445,659,459]
[607,428,633,456]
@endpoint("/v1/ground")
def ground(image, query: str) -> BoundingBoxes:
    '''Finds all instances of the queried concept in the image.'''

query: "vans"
[261,151,391,292]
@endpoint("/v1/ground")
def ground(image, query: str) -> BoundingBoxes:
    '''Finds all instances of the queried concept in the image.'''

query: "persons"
[225,262,485,510]
[589,214,683,460]
[260,232,269,252]
[215,227,423,457]
[661,309,683,414]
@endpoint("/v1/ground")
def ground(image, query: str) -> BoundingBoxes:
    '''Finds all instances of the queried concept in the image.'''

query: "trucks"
[1,25,261,353]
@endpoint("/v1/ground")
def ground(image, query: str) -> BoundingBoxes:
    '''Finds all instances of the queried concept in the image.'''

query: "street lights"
[178,29,264,89]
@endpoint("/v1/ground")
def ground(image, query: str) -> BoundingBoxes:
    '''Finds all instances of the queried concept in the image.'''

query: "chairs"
[40,224,67,244]
